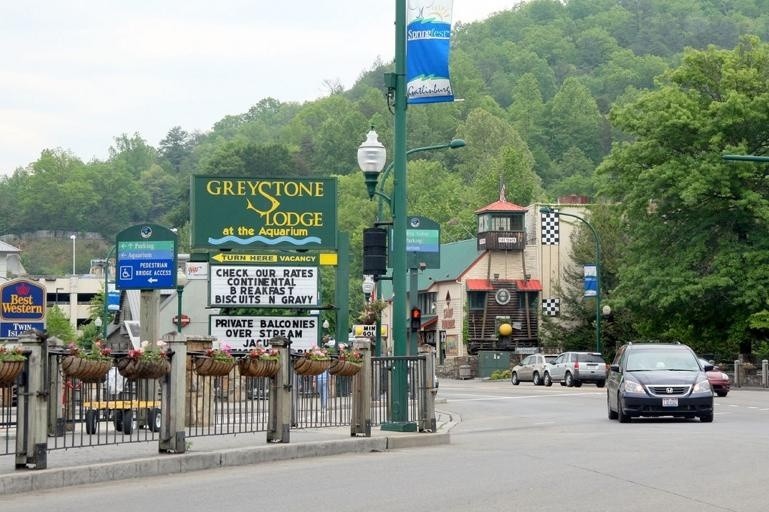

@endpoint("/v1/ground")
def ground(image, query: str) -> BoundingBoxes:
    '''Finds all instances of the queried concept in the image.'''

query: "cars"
[212,378,318,399]
[378,360,439,400]
[509,339,733,425]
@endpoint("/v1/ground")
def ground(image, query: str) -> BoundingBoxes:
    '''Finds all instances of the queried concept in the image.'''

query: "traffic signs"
[113,238,176,290]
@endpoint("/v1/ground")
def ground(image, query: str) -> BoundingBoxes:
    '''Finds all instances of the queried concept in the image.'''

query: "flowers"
[337,350,363,360]
[302,346,327,357]
[244,348,279,358]
[204,345,231,358]
[68,342,111,358]
[1,343,28,360]
[127,348,167,357]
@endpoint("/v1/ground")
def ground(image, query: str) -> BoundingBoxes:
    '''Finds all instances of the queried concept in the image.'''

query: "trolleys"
[75,393,162,433]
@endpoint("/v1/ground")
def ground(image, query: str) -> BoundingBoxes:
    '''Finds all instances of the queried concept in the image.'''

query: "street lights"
[535,208,610,356]
[68,235,77,277]
[94,315,102,335]
[171,267,187,335]
[101,226,178,345]
[350,124,420,430]
[322,314,330,339]
[52,286,66,339]
[363,137,468,363]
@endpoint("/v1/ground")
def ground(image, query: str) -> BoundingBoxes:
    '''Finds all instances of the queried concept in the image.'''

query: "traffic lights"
[408,304,422,333]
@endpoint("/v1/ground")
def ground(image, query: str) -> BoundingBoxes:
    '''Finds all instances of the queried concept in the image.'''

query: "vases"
[330,358,364,376]
[192,356,235,375]
[291,357,331,376]
[1,360,24,385]
[62,356,115,383]
[117,358,171,381]
[237,357,279,377]
